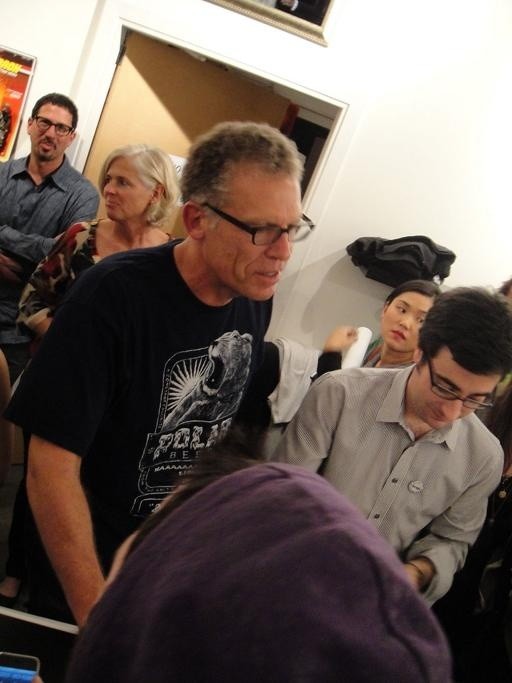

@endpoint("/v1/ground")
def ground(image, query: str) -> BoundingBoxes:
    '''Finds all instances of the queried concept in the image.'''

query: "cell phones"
[0,652,41,683]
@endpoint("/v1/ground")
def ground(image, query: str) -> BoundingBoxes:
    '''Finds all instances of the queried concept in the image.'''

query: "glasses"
[32,116,74,137]
[204,201,316,246]
[427,356,498,411]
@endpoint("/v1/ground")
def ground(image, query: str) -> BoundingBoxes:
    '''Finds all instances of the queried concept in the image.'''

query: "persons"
[269,285,512,612]
[309,278,445,375]
[466,276,512,486]
[10,141,180,343]
[50,447,453,681]
[5,119,315,635]
[2,93,95,296]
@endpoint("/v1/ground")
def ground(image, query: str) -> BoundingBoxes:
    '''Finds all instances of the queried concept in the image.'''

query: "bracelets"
[404,559,426,590]
